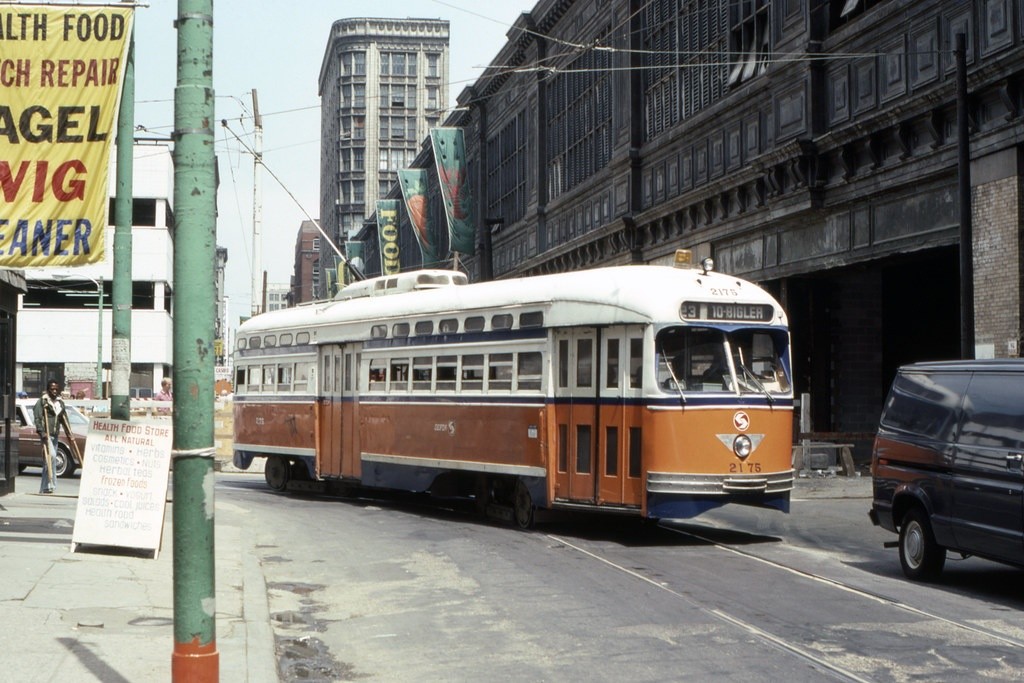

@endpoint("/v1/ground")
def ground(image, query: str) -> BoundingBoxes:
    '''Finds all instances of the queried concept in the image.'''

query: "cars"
[16,398,89,477]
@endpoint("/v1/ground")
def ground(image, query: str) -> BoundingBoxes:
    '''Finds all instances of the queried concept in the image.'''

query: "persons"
[34,381,74,493]
[62,391,85,400]
[19,391,27,399]
[155,377,172,412]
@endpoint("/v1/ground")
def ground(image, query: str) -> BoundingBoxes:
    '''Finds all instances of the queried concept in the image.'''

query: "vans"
[869,358,1024,580]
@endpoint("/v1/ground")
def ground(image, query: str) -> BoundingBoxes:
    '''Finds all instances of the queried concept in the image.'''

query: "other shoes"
[42,488,54,494]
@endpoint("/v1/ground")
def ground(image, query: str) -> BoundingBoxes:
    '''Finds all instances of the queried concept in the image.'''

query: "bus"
[872,372,970,493]
[230,250,797,531]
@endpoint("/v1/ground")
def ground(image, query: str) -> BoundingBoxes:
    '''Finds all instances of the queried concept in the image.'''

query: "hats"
[18,392,28,397]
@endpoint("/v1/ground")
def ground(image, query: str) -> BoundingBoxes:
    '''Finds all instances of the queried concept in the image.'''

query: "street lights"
[53,273,104,399]
